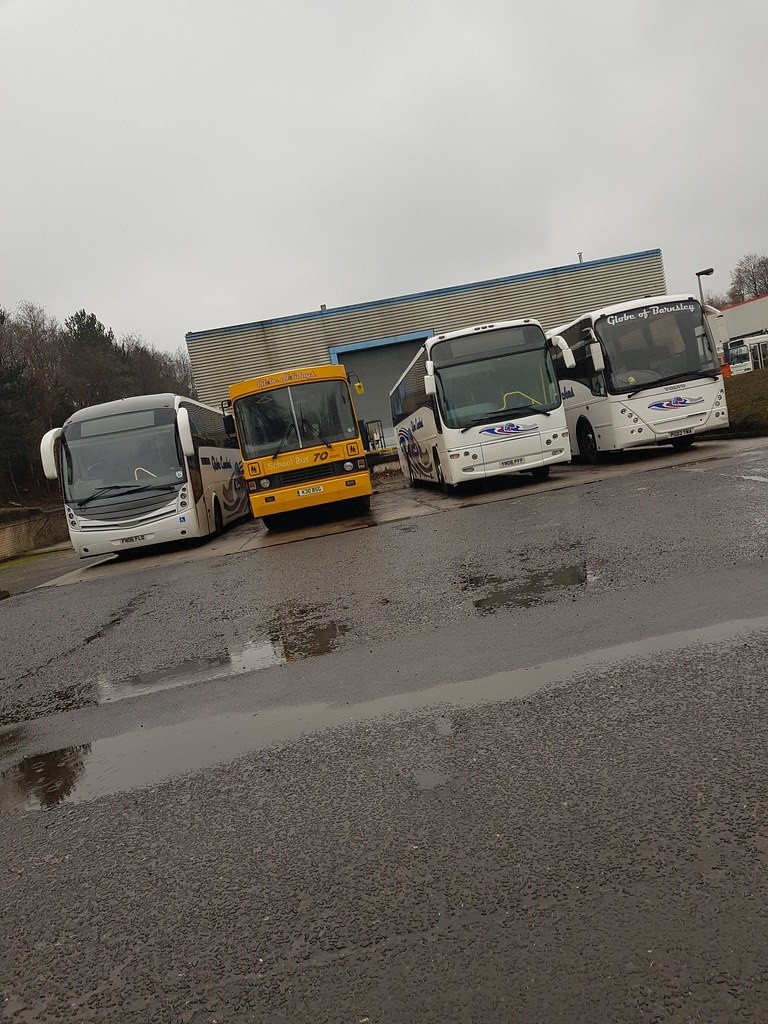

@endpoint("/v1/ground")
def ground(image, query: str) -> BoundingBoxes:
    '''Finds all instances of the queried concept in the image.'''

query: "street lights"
[696,266,714,306]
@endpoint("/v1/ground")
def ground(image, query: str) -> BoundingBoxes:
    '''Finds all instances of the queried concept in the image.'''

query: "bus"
[219,365,375,532]
[544,292,729,464]
[41,394,250,564]
[389,317,576,495]
[724,333,768,376]
